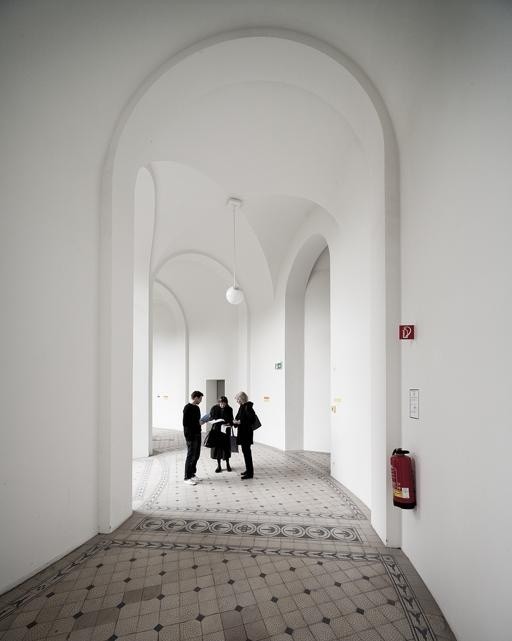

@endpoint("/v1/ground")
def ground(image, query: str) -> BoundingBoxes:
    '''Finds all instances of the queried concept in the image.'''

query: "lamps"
[224,197,246,306]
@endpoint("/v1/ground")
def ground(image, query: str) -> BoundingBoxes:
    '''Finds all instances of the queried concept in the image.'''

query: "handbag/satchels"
[247,407,262,433]
[229,429,239,453]
[203,423,218,448]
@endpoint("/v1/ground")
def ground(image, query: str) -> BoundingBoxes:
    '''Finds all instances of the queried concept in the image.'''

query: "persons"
[233,391,254,481]
[205,397,235,473]
[181,391,205,487]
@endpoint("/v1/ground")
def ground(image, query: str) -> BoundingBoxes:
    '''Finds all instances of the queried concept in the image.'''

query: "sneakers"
[183,474,203,485]
[241,471,254,480]
[215,465,232,473]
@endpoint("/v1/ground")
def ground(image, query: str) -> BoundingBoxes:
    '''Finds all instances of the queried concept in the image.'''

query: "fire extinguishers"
[390,448,416,510]
